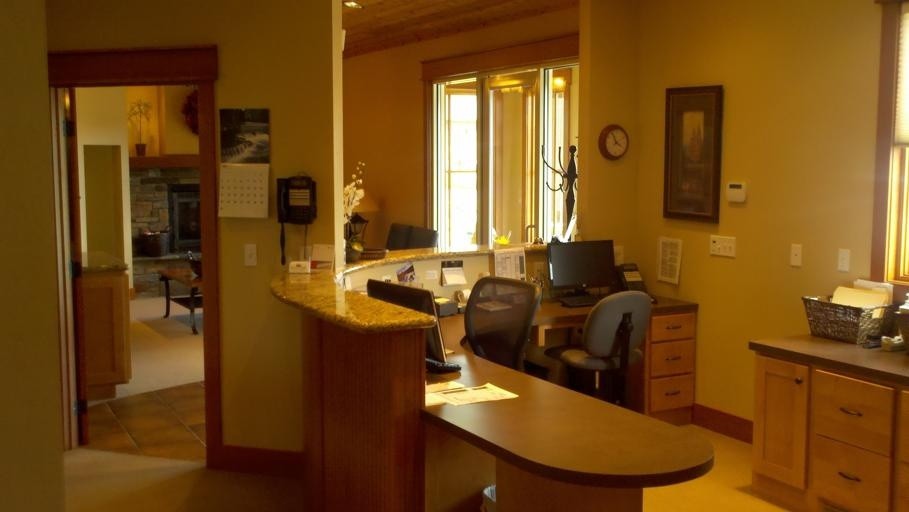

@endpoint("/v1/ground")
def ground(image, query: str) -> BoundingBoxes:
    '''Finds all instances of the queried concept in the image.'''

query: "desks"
[420,292,714,512]
[158,268,203,335]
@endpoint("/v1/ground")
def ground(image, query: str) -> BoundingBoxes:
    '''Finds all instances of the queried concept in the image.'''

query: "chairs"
[386,222,439,252]
[464,276,539,378]
[545,290,652,405]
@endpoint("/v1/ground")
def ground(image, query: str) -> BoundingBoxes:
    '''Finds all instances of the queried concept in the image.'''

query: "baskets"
[801,295,896,344]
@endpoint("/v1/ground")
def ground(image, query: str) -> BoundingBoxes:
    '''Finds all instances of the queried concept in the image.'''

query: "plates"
[360,248,387,260]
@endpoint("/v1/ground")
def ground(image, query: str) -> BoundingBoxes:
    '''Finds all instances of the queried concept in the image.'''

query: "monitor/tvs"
[545,239,618,295]
[365,278,450,364]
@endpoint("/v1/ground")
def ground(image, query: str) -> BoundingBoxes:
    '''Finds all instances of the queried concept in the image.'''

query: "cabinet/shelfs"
[810,362,909,512]
[78,272,132,401]
[752,354,810,512]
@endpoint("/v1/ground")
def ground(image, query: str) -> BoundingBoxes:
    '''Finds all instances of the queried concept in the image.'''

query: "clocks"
[599,125,629,160]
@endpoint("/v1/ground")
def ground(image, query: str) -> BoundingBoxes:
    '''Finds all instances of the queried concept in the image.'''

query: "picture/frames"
[663,85,724,224]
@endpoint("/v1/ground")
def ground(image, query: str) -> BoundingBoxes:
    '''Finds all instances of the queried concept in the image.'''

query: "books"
[830,278,894,319]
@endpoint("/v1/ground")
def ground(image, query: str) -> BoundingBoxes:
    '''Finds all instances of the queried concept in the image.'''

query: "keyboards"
[427,359,461,374]
[557,294,605,309]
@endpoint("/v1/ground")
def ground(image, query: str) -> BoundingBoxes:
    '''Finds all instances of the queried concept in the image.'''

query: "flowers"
[343,160,366,225]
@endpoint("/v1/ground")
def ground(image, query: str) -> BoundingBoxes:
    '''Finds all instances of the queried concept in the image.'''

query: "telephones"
[607,262,648,292]
[277,176,317,227]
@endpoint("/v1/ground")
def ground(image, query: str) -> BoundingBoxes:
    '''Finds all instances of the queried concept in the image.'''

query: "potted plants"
[128,96,153,157]
